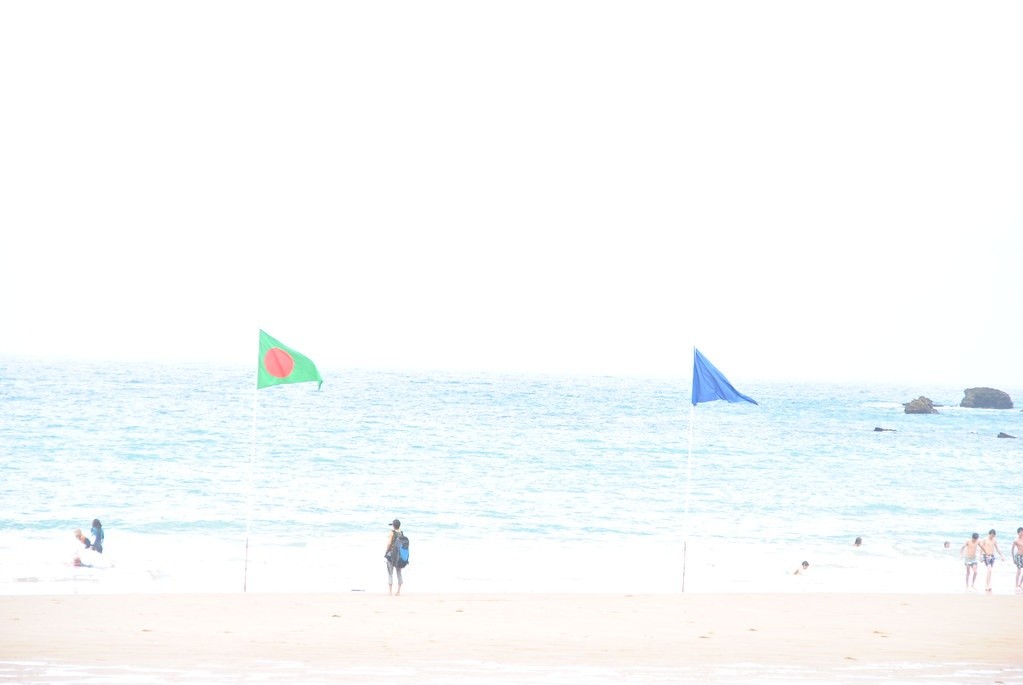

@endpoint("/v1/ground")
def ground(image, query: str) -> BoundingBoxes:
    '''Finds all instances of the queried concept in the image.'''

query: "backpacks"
[390,530,410,568]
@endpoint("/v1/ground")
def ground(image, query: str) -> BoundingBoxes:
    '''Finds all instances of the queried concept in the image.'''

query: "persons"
[74,528,90,552]
[384,519,404,596]
[87,518,105,554]
[1011,527,1023,590]
[980,528,1005,591]
[960,532,988,589]
